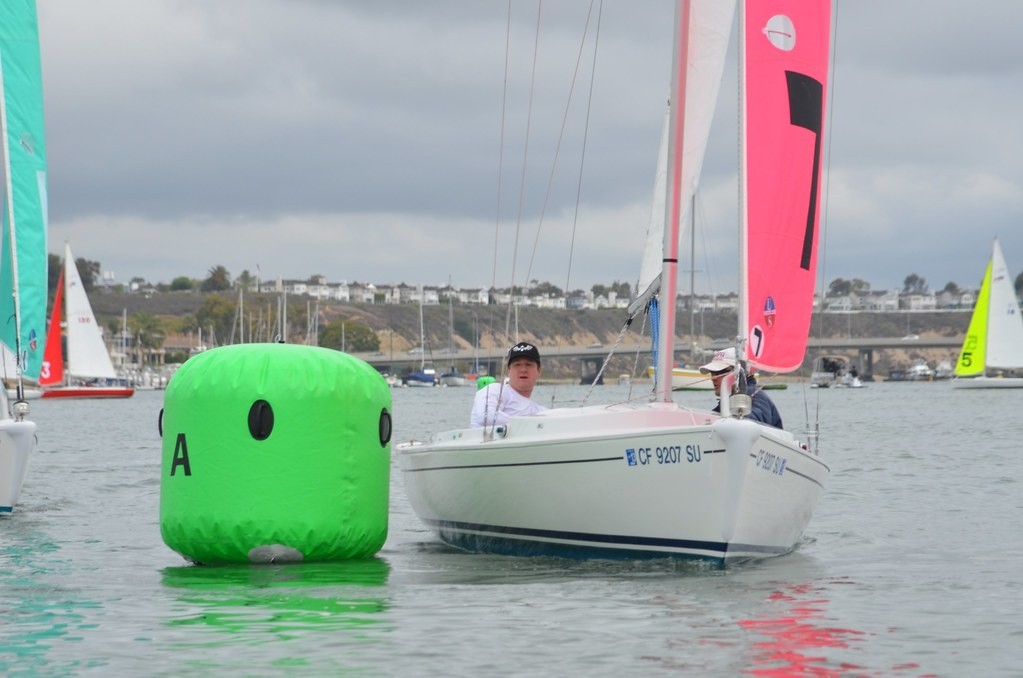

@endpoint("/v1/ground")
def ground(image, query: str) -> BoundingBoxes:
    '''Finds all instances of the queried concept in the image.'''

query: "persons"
[471,341,550,426]
[824,360,858,381]
[699,346,784,431]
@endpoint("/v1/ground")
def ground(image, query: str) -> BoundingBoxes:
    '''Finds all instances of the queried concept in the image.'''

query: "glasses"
[709,368,731,375]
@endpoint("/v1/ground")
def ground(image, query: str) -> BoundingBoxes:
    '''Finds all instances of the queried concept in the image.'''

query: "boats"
[810,352,866,389]
[882,354,956,381]
[644,363,789,392]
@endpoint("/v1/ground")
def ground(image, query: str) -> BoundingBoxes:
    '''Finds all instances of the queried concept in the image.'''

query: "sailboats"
[31,241,139,400]
[0,0,53,517]
[948,238,1023,389]
[98,280,468,392]
[389,1,835,559]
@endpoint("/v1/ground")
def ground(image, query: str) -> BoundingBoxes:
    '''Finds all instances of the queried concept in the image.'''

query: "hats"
[699,348,737,375]
[508,341,541,365]
